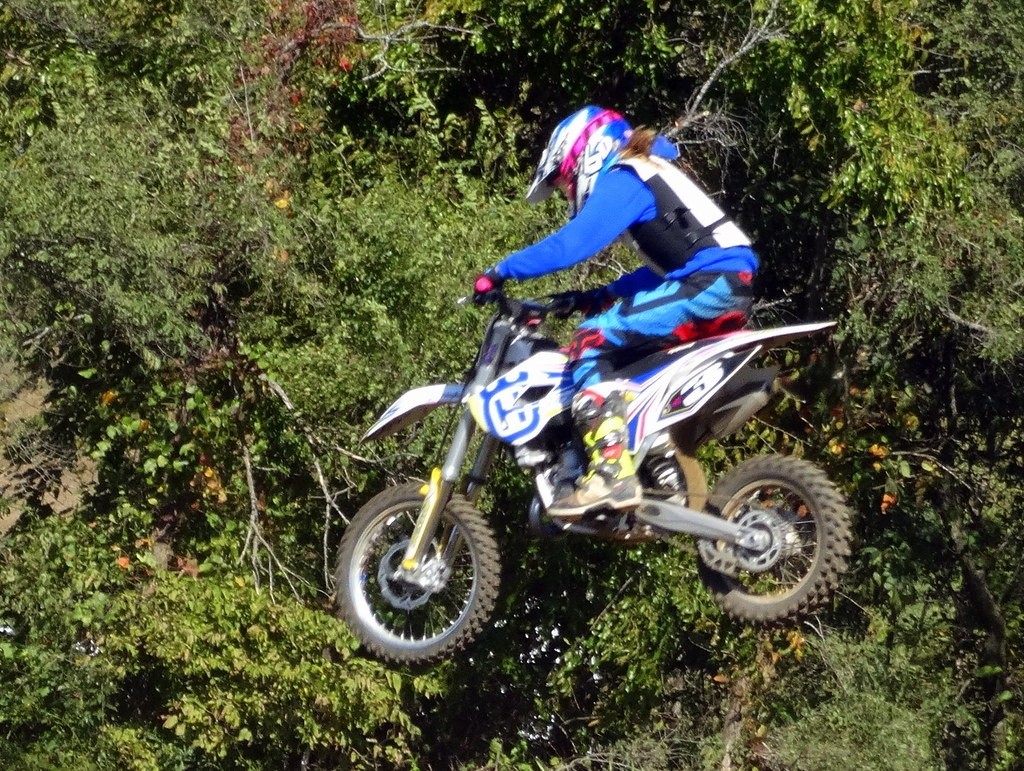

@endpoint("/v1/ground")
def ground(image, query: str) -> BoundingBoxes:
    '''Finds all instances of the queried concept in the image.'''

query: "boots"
[545,381,646,516]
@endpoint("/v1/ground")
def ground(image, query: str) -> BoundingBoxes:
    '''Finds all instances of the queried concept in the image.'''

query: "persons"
[471,106,762,520]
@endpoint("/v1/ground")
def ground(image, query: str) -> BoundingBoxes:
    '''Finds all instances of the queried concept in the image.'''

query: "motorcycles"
[332,274,856,676]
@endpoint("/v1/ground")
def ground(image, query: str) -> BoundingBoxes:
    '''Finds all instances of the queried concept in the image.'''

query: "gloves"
[472,266,505,307]
[554,287,581,320]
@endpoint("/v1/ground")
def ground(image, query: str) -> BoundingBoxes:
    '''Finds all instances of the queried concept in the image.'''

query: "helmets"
[523,105,637,222]
